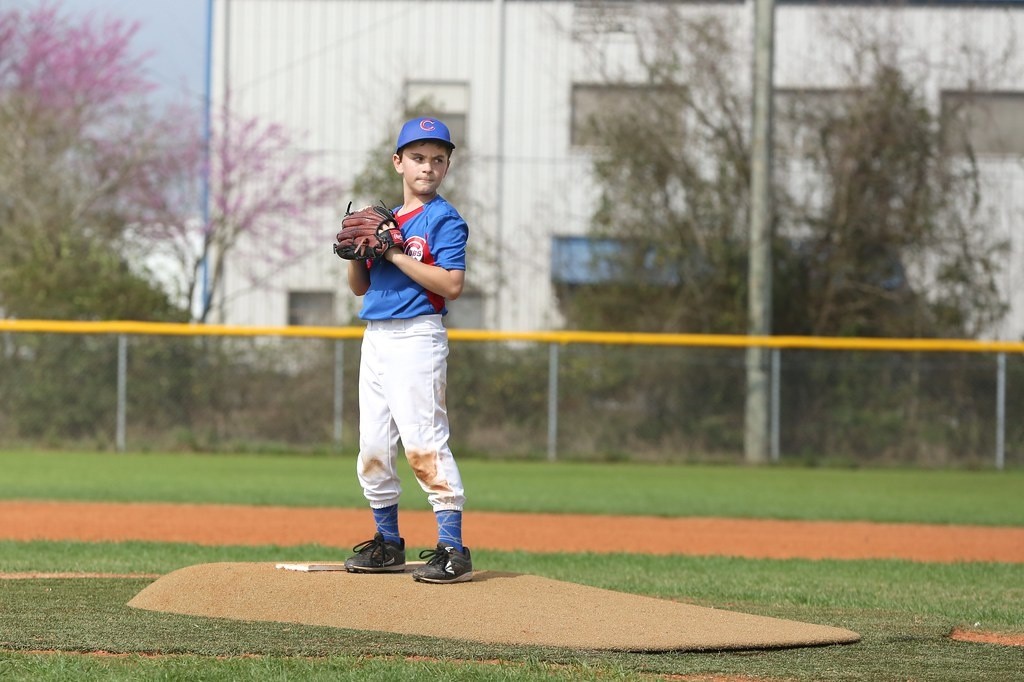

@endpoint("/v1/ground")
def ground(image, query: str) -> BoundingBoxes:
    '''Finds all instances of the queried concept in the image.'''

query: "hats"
[396,116,456,159]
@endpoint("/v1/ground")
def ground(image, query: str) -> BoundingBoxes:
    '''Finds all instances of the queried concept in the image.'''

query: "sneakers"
[413,541,473,583]
[344,532,406,573]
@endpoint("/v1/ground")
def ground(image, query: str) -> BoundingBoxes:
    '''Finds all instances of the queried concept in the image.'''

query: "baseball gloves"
[336,208,403,266]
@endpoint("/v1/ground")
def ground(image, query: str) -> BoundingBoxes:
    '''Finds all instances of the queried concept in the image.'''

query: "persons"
[335,117,473,584]
